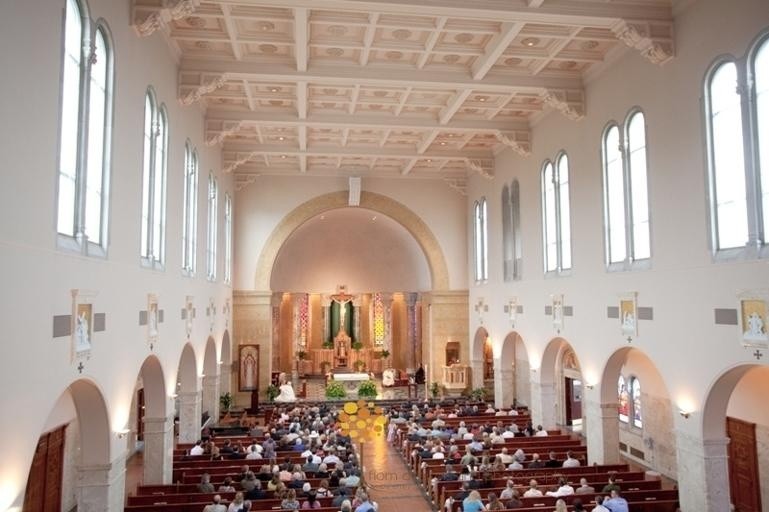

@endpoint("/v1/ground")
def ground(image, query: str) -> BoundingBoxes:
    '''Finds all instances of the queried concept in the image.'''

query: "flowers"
[326,383,347,397]
[358,382,376,397]
[220,393,232,407]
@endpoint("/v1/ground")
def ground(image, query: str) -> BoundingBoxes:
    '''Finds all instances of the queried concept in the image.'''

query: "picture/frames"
[238,343,260,392]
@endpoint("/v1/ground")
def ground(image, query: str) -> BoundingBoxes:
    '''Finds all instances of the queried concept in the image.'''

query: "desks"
[334,374,369,389]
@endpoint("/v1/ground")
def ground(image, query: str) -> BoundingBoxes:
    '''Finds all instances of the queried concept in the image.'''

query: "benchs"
[126,407,365,512]
[387,399,678,511]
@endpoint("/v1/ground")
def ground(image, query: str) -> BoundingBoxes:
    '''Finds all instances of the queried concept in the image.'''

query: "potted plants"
[322,340,332,348]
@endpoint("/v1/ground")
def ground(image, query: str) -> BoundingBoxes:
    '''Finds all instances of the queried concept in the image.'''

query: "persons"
[384,399,629,512]
[190,400,375,512]
[274,372,295,402]
[415,363,426,384]
